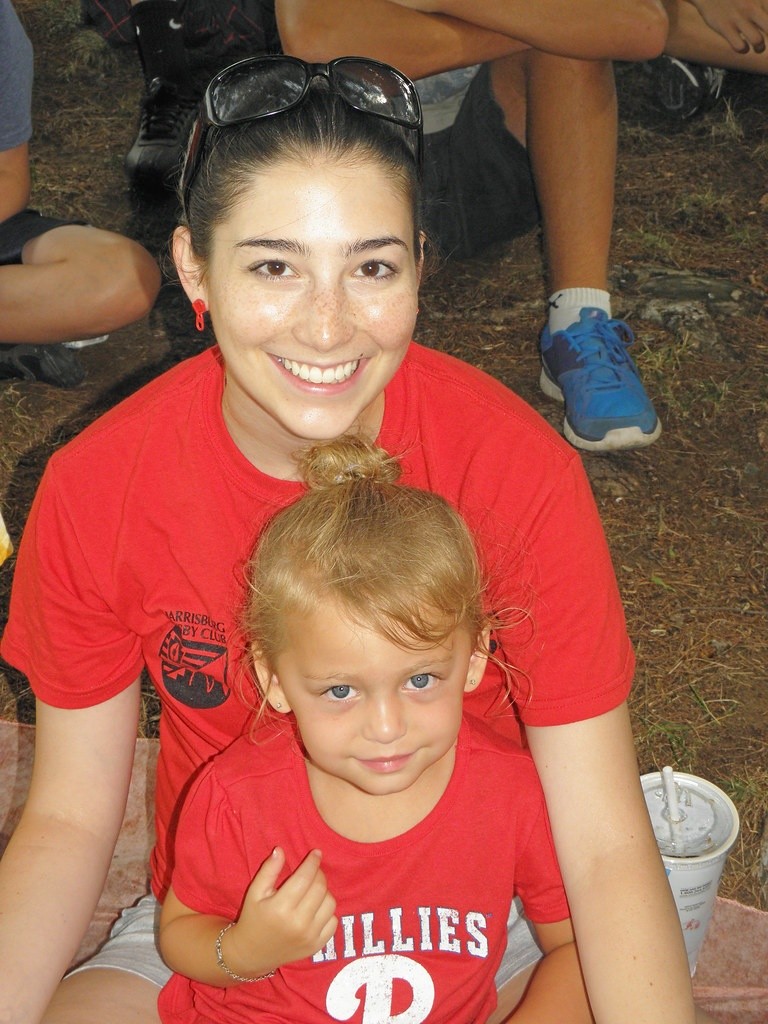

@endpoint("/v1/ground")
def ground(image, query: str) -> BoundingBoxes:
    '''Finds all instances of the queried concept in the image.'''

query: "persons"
[639,0,768,119]
[0,0,162,389]
[157,434,593,1024]
[84,0,275,188]
[275,0,668,452]
[0,83,714,1024]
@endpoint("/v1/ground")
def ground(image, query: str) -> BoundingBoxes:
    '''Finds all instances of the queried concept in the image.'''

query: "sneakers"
[125,71,210,192]
[0,333,87,390]
[538,307,663,452]
[614,53,725,119]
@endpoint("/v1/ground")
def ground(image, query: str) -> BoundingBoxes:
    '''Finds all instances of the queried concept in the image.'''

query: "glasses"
[183,53,425,228]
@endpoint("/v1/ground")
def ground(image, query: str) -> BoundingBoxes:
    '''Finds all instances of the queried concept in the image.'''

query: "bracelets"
[216,923,276,982]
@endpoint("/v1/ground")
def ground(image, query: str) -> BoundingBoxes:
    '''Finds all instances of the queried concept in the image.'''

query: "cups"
[636,774,740,976]
